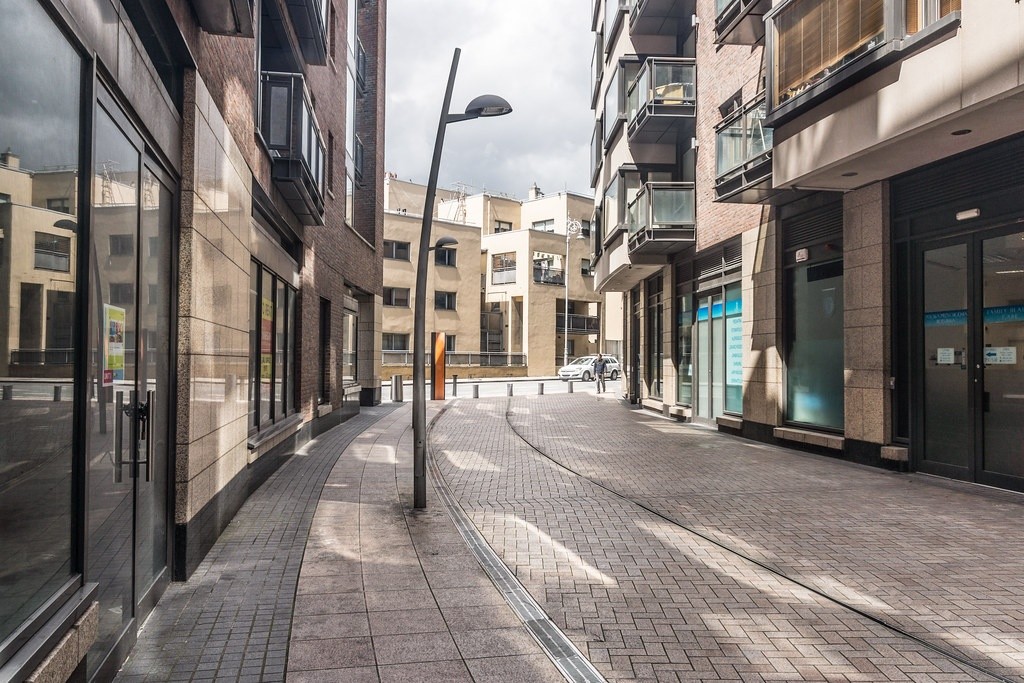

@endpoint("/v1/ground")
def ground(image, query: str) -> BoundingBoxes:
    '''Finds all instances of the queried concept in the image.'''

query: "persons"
[594,354,606,394]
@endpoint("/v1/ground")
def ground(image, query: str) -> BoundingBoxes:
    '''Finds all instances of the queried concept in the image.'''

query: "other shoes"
[596,390,600,394]
[603,388,605,393]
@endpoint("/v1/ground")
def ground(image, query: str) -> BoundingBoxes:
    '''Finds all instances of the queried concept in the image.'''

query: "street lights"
[411,48,514,510]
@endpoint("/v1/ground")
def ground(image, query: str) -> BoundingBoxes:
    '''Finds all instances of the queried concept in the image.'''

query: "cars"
[558,352,622,382]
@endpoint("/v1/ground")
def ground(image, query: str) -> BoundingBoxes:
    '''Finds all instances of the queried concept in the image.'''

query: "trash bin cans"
[391,375,404,402]
